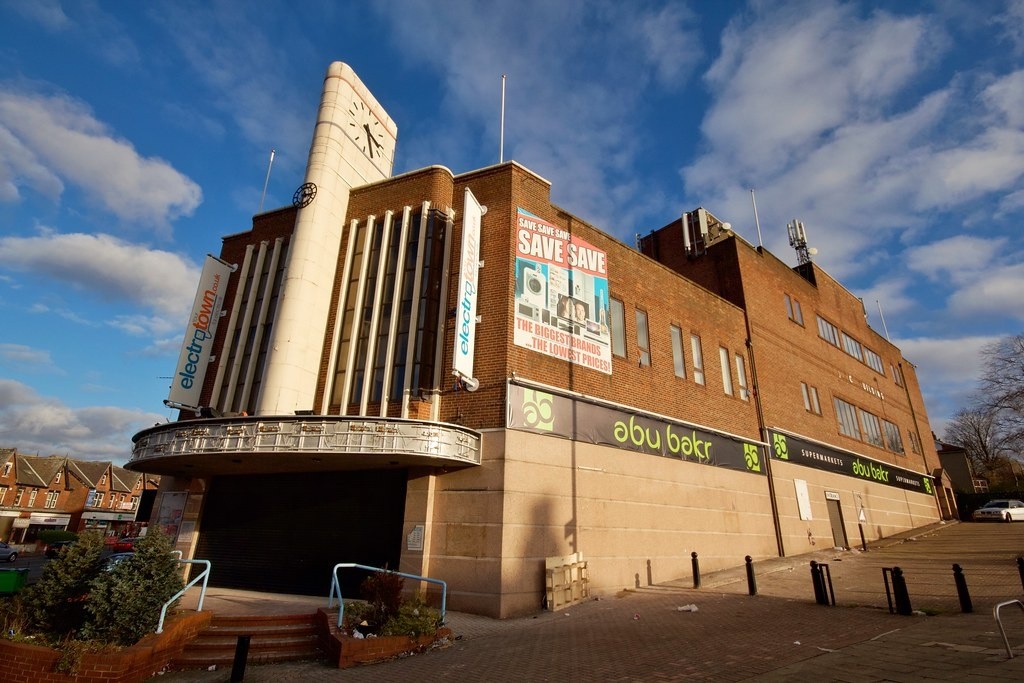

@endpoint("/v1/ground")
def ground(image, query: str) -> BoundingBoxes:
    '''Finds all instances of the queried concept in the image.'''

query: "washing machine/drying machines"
[523,267,547,310]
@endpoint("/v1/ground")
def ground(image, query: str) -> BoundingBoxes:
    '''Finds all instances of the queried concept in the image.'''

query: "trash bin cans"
[0,569,30,597]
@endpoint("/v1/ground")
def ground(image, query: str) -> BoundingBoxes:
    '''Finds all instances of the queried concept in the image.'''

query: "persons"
[560,297,586,324]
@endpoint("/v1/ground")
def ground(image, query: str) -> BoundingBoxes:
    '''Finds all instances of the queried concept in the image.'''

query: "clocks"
[347,98,385,160]
[292,182,317,208]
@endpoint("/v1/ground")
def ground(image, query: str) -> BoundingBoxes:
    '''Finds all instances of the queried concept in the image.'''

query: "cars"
[0,541,19,563]
[45,540,76,559]
[113,536,145,553]
[971,498,1024,523]
